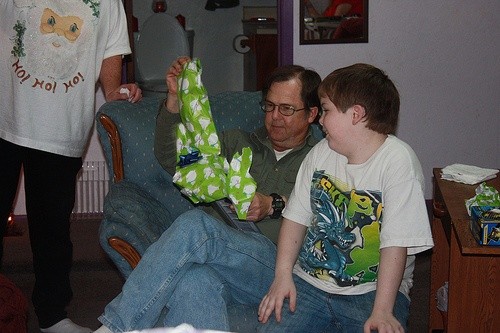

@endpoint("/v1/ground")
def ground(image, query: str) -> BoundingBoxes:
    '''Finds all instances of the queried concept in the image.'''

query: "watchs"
[267,193,286,220]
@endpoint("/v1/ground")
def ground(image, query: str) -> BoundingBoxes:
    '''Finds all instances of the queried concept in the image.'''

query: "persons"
[0,0,142,333]
[256,63,435,333]
[92,54,325,333]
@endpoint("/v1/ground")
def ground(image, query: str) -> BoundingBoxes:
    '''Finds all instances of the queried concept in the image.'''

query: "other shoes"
[40,318,94,333]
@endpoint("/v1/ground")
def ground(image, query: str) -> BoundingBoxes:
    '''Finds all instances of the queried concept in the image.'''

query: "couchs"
[96,92,327,283]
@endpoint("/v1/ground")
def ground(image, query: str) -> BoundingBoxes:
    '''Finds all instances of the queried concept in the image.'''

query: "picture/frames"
[299,0,369,45]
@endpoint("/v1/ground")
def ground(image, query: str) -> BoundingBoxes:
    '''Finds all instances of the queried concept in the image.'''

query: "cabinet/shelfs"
[428,168,500,333]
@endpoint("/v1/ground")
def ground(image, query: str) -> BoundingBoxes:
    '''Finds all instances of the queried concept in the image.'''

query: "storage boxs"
[469,205,500,246]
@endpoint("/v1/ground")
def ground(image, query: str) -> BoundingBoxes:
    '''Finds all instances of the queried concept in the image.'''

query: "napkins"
[441,163,499,185]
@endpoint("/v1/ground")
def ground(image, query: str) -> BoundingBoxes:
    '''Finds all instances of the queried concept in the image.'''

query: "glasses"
[258,100,308,116]
